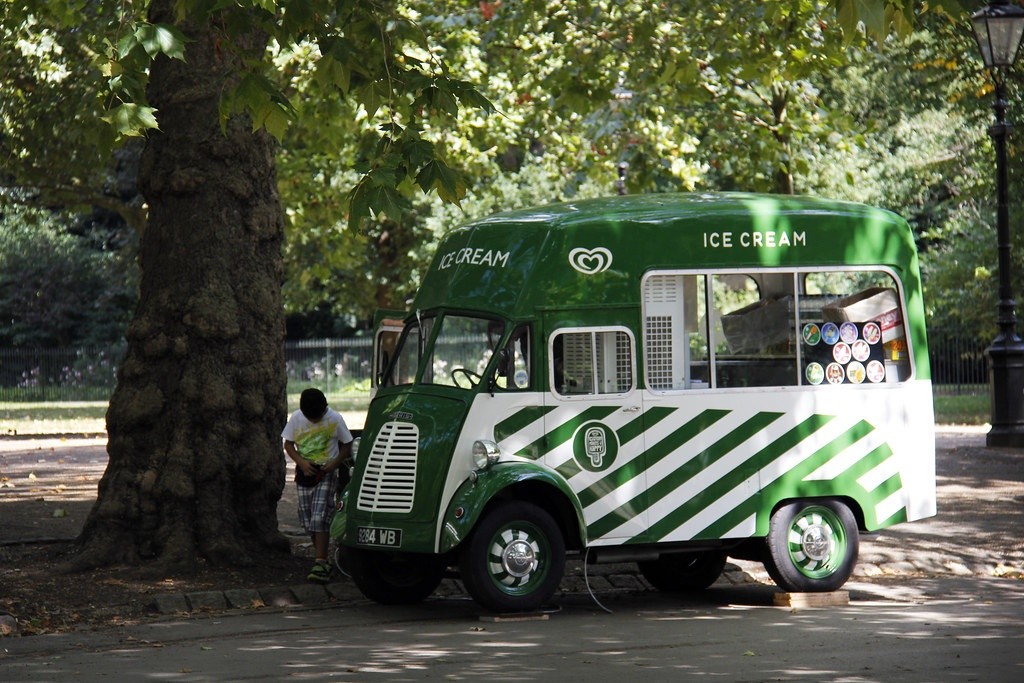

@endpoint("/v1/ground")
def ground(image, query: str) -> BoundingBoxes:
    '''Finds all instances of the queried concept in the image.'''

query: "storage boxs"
[821,287,904,343]
[720,293,792,353]
[883,338,907,362]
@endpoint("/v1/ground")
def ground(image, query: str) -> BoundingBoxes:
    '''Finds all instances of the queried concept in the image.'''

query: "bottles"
[513,337,529,388]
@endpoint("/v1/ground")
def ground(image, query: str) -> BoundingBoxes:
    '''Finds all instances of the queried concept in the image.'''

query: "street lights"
[966,0,1024,446]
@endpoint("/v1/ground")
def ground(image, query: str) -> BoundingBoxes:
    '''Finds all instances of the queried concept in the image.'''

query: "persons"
[281,388,352,582]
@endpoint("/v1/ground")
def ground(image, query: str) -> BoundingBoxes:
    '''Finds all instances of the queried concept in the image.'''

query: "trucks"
[326,187,937,615]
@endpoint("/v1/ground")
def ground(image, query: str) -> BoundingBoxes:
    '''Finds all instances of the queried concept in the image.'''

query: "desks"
[690,359,804,387]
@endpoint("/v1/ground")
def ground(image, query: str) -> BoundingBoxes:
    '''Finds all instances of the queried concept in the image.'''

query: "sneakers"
[307,561,338,584]
